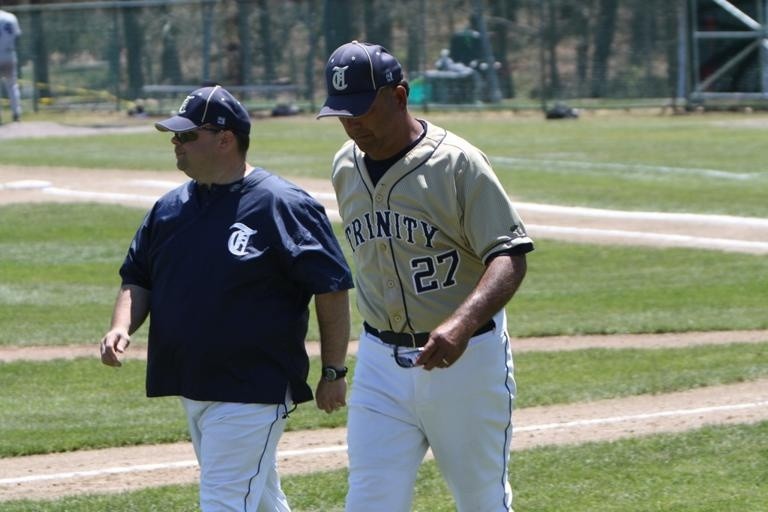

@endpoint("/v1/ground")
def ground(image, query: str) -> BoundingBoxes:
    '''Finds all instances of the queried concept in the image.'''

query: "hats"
[316,40,403,119]
[154,84,250,131]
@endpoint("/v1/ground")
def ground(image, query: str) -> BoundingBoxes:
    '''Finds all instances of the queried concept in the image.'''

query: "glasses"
[173,132,197,142]
[394,319,423,367]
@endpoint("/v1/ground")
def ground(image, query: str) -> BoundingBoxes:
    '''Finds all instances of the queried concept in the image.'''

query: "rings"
[442,358,450,366]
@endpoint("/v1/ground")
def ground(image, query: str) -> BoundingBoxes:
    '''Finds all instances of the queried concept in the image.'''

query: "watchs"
[322,365,348,382]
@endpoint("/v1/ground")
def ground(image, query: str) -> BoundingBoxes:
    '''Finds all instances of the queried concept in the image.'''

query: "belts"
[363,319,495,346]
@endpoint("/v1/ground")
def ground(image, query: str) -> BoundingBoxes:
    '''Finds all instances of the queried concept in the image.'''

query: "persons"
[0,9,24,123]
[97,84,356,510]
[314,36,536,511]
